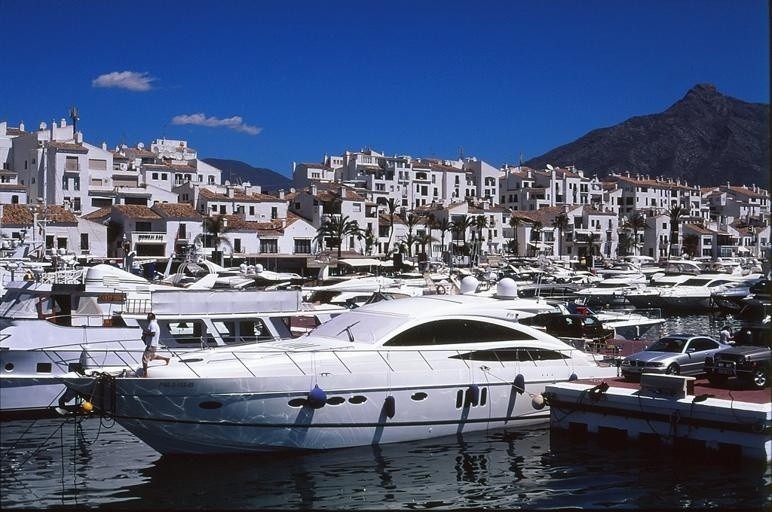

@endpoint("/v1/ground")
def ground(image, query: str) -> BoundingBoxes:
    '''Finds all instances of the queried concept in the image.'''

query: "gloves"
[666,253,771,315]
[1,414,163,511]
[639,310,742,349]
[26,296,626,456]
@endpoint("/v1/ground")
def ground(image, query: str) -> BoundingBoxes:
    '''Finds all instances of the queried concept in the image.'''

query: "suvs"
[702,325,771,390]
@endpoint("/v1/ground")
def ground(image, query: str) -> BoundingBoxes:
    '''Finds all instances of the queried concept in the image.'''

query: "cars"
[127,442,612,509]
[620,333,732,380]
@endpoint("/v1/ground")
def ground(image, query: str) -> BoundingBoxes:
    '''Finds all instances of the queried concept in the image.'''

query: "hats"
[127,442,612,509]
[620,333,732,380]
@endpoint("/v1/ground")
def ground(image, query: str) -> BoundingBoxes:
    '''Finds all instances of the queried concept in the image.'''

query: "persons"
[720,326,736,346]
[142,313,170,378]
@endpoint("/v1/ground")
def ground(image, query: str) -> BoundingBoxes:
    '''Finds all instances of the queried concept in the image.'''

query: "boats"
[1,414,163,511]
[666,253,771,315]
[46,294,626,456]
[0,248,666,412]
[26,296,626,456]
[639,310,742,349]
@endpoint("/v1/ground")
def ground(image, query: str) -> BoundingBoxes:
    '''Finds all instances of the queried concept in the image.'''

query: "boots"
[702,325,771,390]
